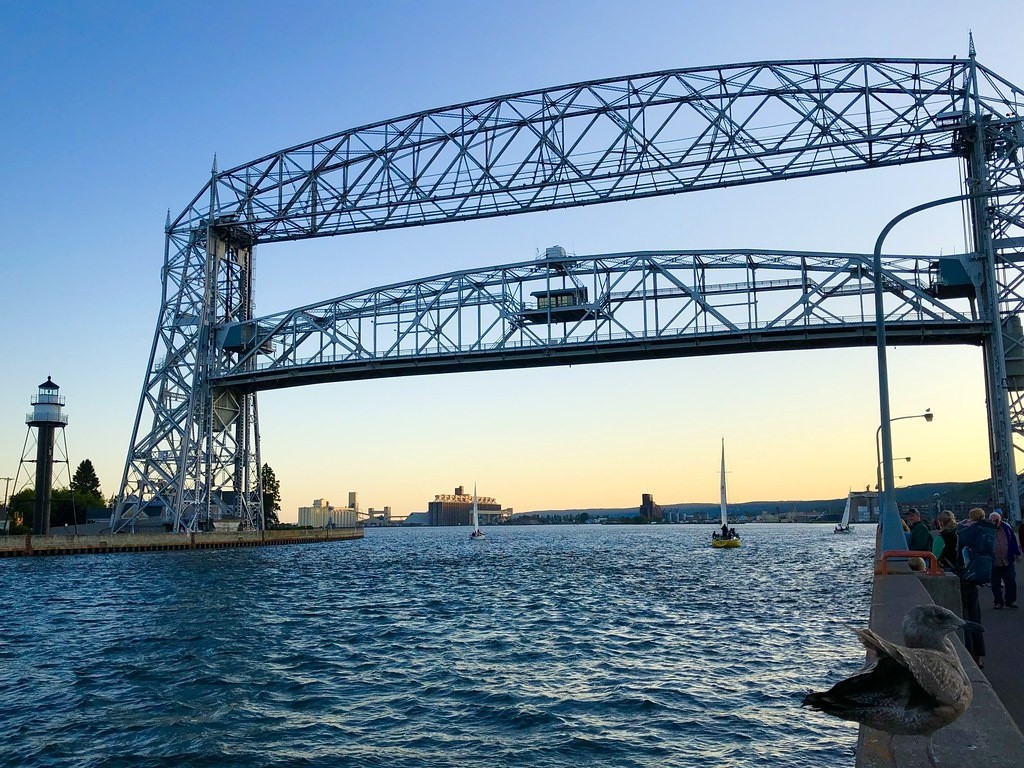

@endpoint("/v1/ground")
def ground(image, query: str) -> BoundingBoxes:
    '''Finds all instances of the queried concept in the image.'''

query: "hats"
[994,509,1003,515]
[903,508,920,514]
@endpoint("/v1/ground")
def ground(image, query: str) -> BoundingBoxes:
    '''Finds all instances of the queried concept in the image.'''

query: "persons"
[957,506,993,587]
[905,506,932,569]
[900,518,911,546]
[1017,522,1024,553]
[930,510,985,669]
[992,508,1013,528]
[928,519,941,540]
[712,524,740,541]
[988,511,1023,609]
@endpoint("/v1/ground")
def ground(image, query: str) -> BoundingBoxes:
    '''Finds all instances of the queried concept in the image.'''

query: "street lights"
[874,413,935,505]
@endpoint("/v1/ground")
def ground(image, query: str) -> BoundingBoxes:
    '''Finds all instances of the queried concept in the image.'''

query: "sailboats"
[711,437,742,548]
[470,482,486,540]
[833,487,854,534]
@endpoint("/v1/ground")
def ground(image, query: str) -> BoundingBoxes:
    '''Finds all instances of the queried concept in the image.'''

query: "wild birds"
[800,604,986,768]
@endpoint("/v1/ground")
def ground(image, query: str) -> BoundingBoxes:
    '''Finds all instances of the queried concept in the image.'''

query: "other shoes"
[1004,604,1019,609]
[993,603,1004,609]
